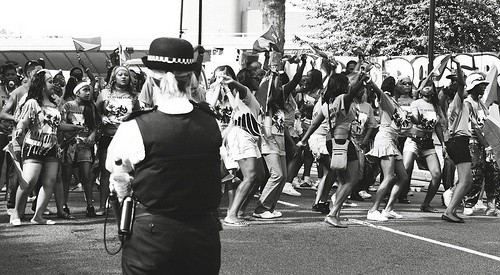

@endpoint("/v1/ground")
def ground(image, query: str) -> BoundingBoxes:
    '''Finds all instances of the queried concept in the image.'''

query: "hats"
[141,37,198,71]
[395,75,410,86]
[465,73,489,92]
[446,69,467,79]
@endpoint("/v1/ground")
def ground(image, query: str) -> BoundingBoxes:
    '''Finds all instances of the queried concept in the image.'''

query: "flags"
[289,33,305,47]
[306,41,322,53]
[253,25,283,54]
[72,36,102,53]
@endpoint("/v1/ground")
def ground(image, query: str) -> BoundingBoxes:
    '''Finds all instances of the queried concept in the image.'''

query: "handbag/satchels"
[329,138,350,170]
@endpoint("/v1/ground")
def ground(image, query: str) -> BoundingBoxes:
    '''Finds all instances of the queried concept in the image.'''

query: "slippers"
[223,215,257,226]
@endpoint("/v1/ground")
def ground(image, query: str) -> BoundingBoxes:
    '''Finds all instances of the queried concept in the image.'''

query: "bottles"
[110,157,133,204]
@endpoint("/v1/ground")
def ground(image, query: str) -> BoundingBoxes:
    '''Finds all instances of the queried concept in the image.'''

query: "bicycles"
[456,130,495,210]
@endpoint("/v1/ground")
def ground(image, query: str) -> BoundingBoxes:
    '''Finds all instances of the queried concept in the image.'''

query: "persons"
[105,37,223,275]
[0,53,500,228]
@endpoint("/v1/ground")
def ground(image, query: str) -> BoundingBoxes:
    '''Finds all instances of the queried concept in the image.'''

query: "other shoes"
[9,211,21,226]
[32,206,50,214]
[350,186,377,201]
[86,206,105,216]
[57,204,74,219]
[420,204,439,213]
[367,210,388,221]
[399,197,410,204]
[464,206,497,216]
[381,209,402,218]
[408,190,414,196]
[441,188,454,208]
[442,212,465,224]
[324,215,348,228]
[300,182,318,188]
[253,210,282,218]
[31,218,56,224]
[282,182,302,197]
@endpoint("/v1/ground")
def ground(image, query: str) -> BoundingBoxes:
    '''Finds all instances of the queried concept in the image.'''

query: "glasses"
[471,77,485,84]
[402,82,413,86]
